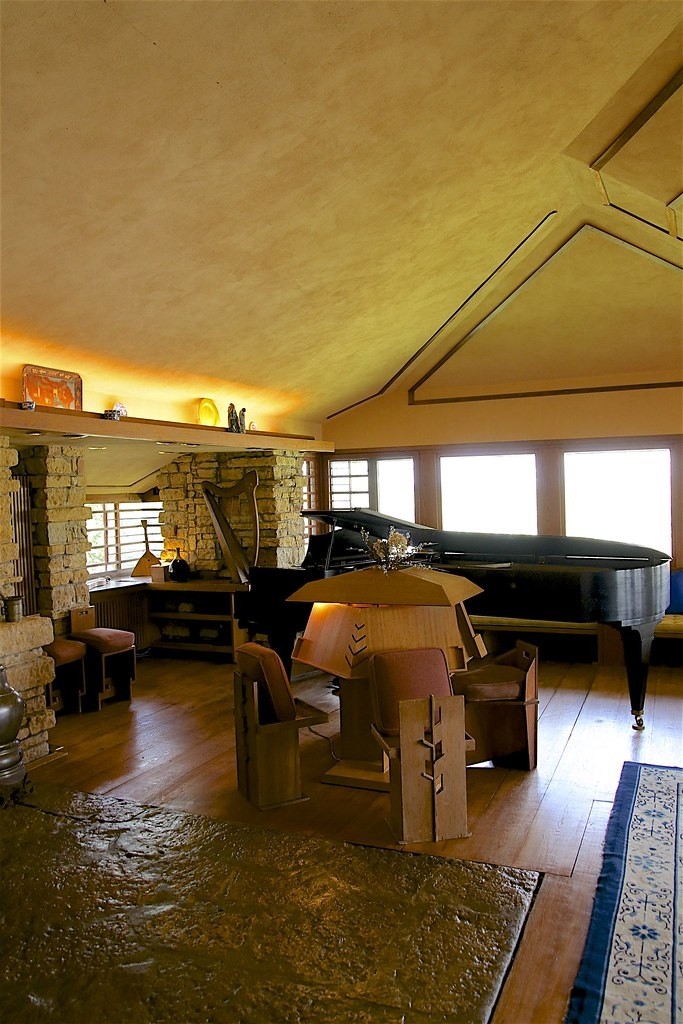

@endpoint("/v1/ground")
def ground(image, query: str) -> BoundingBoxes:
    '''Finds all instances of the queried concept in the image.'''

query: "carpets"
[564,761,683,1024]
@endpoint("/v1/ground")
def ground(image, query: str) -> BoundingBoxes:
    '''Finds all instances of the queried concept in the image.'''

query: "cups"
[1,596,22,622]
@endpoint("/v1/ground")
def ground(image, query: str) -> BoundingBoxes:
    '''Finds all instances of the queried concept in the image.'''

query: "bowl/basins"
[19,401,36,410]
[104,410,119,420]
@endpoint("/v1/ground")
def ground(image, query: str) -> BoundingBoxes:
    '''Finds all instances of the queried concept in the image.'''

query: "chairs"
[41,611,86,713]
[67,604,136,711]
[229,630,539,846]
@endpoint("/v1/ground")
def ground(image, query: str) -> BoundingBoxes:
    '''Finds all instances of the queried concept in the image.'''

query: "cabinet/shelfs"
[121,576,248,663]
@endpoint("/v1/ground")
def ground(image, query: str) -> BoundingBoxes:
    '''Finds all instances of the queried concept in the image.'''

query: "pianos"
[233,505,673,729]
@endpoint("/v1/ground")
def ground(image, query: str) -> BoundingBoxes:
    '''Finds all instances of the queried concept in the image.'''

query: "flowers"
[361,527,439,577]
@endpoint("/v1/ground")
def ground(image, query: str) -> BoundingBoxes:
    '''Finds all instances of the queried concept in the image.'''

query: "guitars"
[132,520,161,576]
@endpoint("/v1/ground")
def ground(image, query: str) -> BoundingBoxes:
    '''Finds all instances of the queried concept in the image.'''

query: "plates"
[113,403,127,416]
[196,398,220,425]
[249,422,258,430]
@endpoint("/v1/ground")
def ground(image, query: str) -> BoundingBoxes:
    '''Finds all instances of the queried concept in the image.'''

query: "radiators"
[90,583,164,651]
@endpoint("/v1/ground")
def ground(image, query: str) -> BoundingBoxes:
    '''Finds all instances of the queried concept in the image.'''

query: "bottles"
[168,547,191,583]
